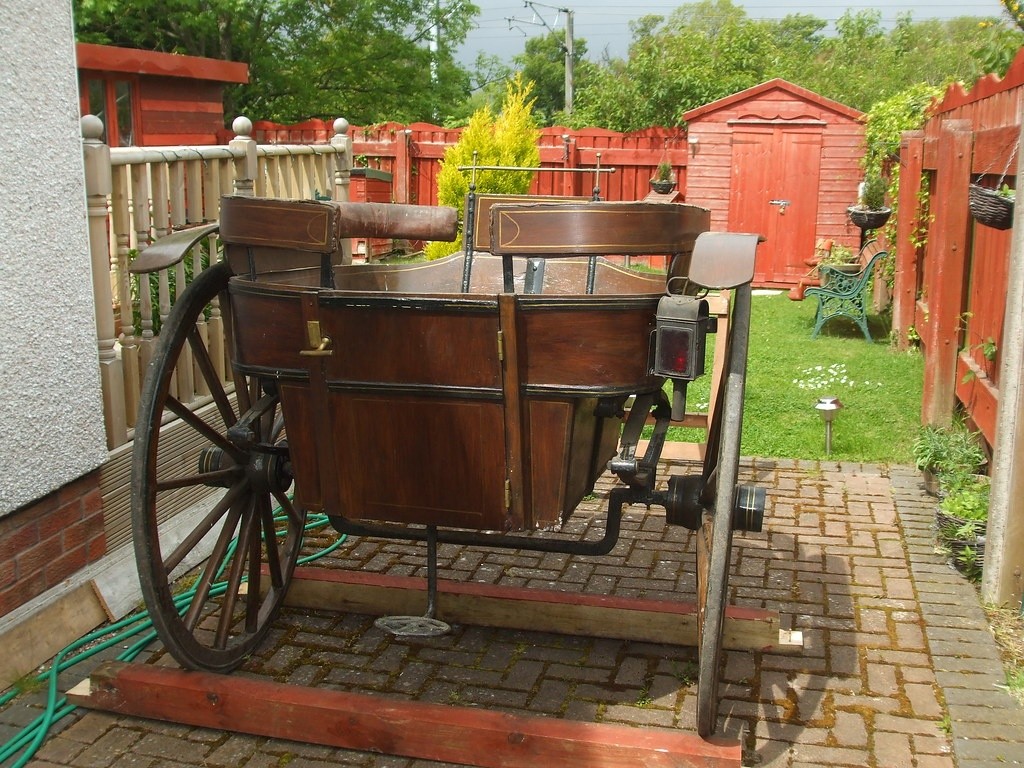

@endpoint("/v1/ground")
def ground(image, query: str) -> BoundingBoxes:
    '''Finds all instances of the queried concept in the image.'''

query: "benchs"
[804,240,886,343]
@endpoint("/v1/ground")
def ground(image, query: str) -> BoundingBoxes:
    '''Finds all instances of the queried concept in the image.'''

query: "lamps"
[812,397,844,422]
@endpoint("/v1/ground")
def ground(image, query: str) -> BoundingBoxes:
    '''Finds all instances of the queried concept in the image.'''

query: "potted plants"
[912,402,991,583]
[648,153,678,195]
[969,181,1015,230]
[847,155,893,229]
